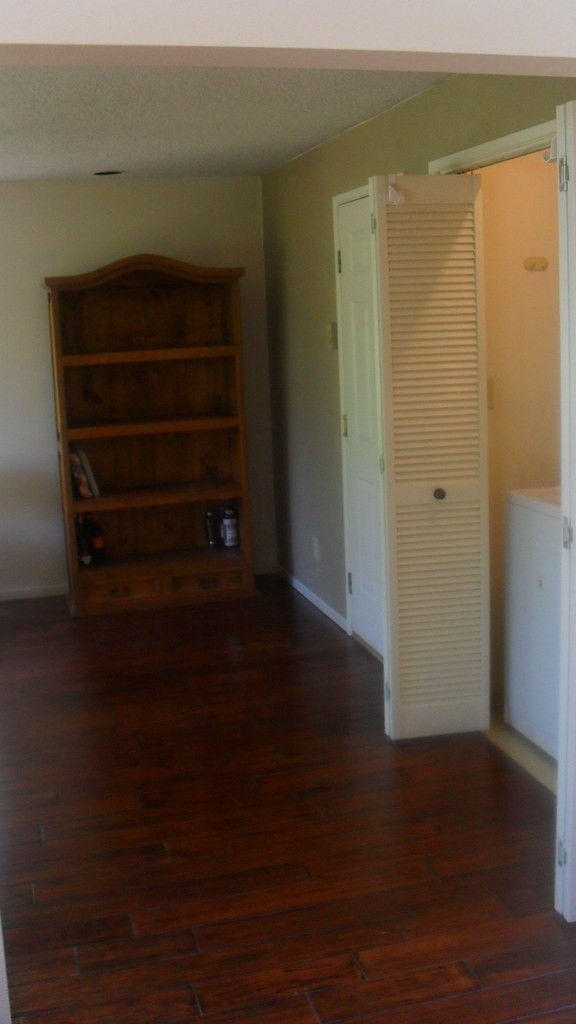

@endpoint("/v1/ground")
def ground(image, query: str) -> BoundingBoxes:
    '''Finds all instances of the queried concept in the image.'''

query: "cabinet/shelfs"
[46,253,263,617]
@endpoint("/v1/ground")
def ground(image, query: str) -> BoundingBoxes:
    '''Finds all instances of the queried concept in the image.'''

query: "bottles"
[204,512,219,546]
[221,507,240,548]
[90,512,106,565]
[76,513,92,568]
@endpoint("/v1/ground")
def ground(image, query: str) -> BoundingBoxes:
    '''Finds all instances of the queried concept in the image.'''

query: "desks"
[503,486,560,761]
